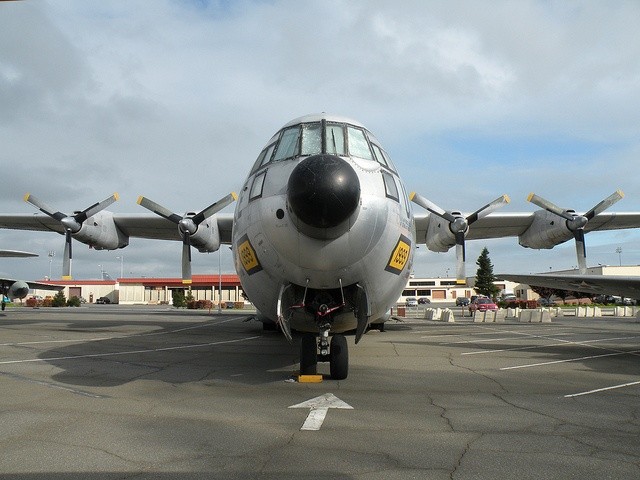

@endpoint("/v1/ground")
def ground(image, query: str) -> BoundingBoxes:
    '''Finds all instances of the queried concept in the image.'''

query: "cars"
[419,298,430,304]
[456,297,468,306]
[405,298,418,308]
[468,298,499,311]
[501,293,517,300]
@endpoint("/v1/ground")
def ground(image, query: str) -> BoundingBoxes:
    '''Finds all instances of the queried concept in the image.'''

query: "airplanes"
[495,273,640,299]
[0,249,39,258]
[0,110,640,379]
[0,278,65,311]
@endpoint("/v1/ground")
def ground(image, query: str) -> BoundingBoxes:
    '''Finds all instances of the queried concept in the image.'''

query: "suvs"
[96,297,110,304]
